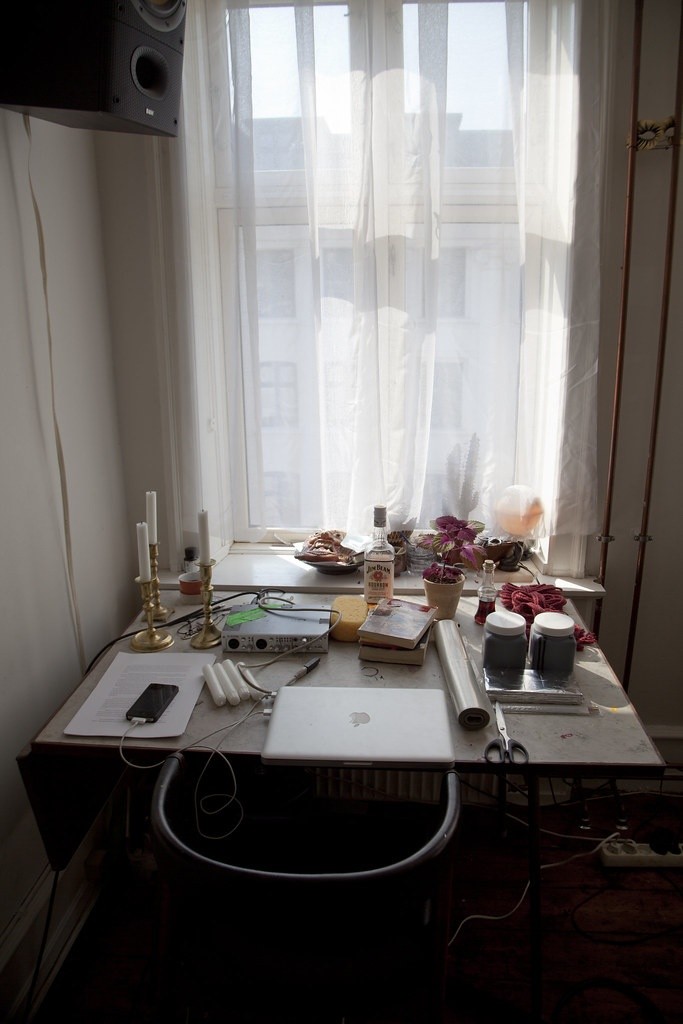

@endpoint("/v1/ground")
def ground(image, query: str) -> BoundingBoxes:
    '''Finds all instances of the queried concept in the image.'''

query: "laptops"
[260,686,455,769]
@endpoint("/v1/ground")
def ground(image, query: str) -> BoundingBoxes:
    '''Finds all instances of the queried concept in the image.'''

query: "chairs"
[150,751,460,1023]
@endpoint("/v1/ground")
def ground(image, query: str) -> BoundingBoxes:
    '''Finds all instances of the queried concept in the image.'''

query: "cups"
[178,571,202,604]
[407,549,437,577]
[393,547,406,577]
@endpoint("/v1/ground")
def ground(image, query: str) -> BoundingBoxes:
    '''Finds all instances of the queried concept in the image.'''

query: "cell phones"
[125,683,179,723]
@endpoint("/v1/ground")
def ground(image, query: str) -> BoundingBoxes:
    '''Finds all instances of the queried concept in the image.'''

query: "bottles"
[474,560,499,625]
[364,506,394,609]
[482,611,528,682]
[528,612,575,677]
[184,547,201,572]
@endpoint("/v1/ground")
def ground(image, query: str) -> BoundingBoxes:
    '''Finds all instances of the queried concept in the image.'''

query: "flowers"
[422,516,484,584]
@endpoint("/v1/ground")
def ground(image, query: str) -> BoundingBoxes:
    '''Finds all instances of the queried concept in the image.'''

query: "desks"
[36,589,666,1024]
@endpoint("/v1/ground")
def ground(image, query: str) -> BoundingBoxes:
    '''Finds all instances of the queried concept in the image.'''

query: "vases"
[424,573,464,619]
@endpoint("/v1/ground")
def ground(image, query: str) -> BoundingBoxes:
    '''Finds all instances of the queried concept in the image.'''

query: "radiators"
[316,767,511,807]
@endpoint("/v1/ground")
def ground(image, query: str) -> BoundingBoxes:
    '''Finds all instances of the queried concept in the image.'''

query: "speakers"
[0,0,187,137]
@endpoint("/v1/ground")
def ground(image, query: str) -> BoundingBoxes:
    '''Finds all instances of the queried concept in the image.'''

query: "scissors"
[484,700,530,766]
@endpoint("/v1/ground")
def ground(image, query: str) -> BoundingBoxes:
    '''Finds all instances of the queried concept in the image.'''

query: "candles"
[145,491,158,545]
[198,511,210,564]
[137,521,152,582]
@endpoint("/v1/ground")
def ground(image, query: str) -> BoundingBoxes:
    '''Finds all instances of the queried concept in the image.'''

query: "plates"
[302,560,364,575]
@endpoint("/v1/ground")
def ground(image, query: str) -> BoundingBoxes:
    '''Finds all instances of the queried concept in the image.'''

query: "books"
[355,596,440,667]
